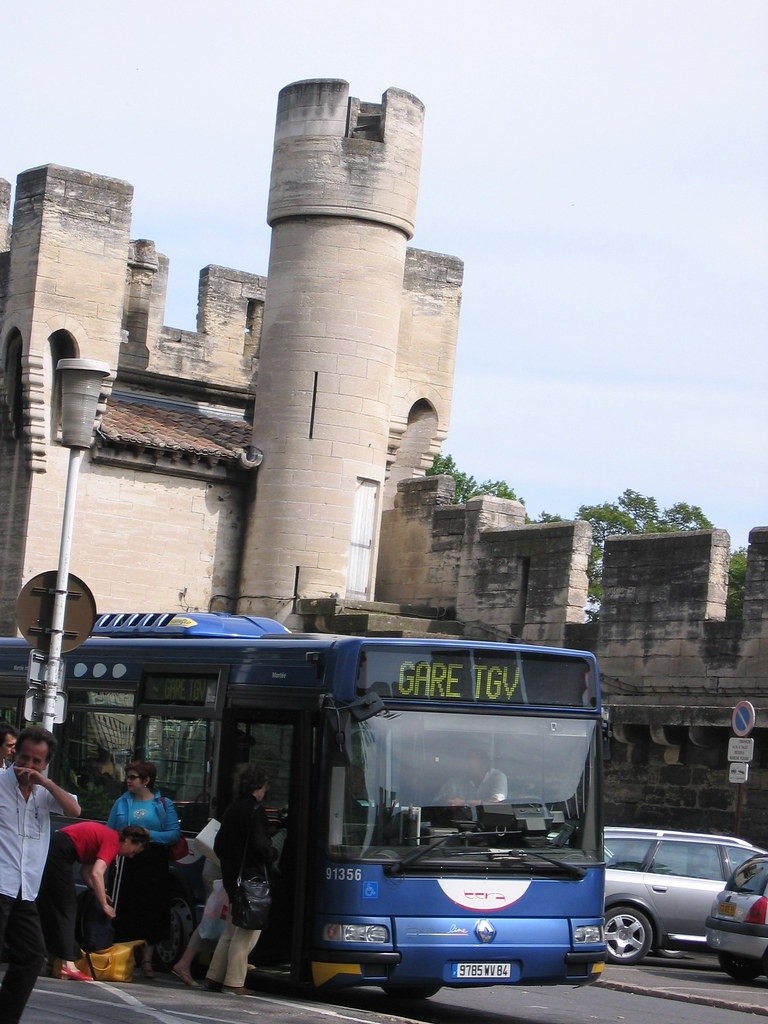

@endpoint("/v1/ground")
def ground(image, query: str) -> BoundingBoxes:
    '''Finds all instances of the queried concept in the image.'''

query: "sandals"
[140,959,154,977]
[172,966,199,986]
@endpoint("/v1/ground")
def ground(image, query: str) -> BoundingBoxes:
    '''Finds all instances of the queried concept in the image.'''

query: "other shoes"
[221,985,255,994]
[201,978,223,992]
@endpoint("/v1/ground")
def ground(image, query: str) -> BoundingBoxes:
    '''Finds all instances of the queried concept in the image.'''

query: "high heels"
[60,966,94,981]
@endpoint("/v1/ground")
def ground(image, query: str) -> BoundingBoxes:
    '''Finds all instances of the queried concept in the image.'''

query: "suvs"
[596,826,767,967]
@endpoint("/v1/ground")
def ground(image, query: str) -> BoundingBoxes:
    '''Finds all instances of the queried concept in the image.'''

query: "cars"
[705,853,768,981]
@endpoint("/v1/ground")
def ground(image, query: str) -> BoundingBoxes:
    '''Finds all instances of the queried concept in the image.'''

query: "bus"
[0,612,608,1002]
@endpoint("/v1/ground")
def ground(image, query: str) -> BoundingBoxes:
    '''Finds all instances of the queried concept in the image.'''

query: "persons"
[432,748,508,806]
[36,761,278,995]
[0,721,81,1024]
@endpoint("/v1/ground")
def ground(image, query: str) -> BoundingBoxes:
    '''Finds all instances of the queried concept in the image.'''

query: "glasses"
[125,775,140,780]
[263,783,270,790]
[16,808,41,840]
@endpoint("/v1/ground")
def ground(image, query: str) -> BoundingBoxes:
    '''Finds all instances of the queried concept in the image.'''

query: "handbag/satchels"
[198,878,229,938]
[160,796,189,860]
[194,819,221,867]
[79,937,145,982]
[232,877,272,931]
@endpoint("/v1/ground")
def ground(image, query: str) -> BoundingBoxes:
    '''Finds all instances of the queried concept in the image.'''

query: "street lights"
[38,357,112,778]
